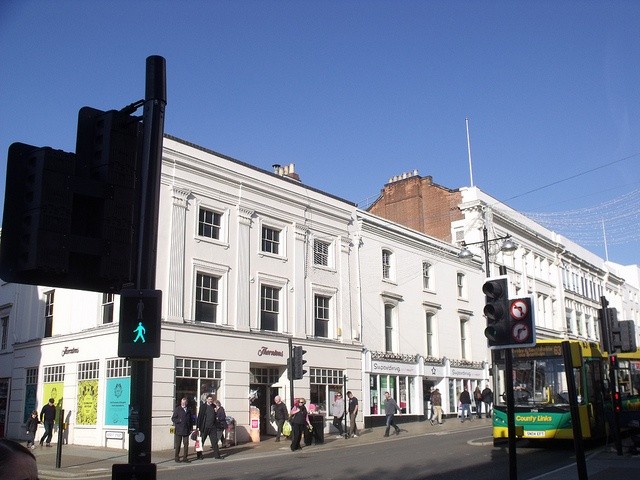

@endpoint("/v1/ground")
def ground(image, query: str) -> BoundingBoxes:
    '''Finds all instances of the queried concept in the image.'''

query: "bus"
[488,340,640,444]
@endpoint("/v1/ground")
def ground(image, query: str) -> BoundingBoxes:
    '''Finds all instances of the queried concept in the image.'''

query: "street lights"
[458,225,518,279]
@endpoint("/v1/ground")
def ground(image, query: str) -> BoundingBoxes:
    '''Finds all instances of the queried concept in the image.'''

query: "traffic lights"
[118,290,161,357]
[614,393,621,411]
[610,356,618,370]
[302,350,307,375]
[482,281,506,344]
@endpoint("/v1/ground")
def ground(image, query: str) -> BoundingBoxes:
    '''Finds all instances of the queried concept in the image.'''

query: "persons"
[40,398,56,447]
[210,400,228,449]
[383,391,403,437]
[290,398,307,451]
[429,389,443,426]
[196,394,224,460]
[473,387,483,419]
[346,391,360,438]
[482,385,493,418]
[460,385,471,423]
[272,395,289,442]
[26,410,42,450]
[332,392,345,439]
[171,397,193,463]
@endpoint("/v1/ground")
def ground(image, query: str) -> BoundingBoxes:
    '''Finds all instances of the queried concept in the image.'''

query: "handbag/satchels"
[195,437,204,452]
[191,431,199,440]
